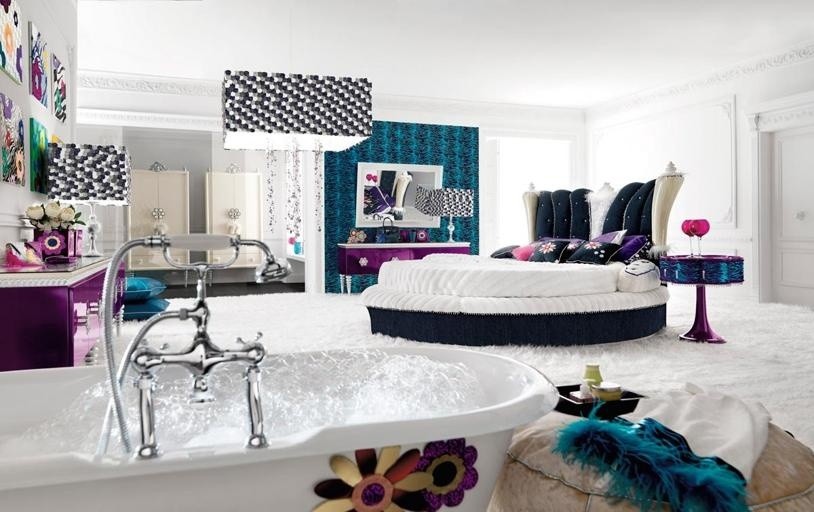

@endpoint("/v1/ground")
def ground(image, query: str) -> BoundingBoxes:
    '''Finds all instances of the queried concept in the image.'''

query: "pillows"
[511,246,533,260]
[566,241,623,265]
[490,245,520,258]
[616,236,652,264]
[591,229,628,245]
[529,237,585,251]
[528,240,570,264]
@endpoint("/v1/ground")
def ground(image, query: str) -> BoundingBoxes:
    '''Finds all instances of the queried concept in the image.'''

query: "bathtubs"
[1,343,558,512]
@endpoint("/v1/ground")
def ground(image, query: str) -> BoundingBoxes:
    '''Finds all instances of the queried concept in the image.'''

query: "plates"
[555,384,649,417]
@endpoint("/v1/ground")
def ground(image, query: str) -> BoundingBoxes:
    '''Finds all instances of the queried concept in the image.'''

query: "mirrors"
[356,160,443,229]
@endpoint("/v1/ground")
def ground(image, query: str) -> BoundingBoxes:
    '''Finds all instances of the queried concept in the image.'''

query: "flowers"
[19,200,86,233]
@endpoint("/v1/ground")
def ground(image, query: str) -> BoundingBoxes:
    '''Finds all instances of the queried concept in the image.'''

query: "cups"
[681,219,696,258]
[689,219,710,258]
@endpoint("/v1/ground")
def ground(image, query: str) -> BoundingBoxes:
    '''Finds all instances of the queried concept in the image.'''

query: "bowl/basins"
[598,386,621,392]
[592,387,625,401]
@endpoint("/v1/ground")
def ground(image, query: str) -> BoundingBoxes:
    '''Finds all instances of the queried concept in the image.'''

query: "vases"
[33,228,74,264]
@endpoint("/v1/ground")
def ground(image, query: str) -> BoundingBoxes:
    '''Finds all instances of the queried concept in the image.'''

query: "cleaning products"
[569,384,594,403]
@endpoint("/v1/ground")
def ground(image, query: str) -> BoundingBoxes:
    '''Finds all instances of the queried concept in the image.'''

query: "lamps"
[48,144,133,257]
[217,69,373,251]
[415,184,474,243]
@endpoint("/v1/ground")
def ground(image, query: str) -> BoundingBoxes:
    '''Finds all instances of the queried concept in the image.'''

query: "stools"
[482,391,814,512]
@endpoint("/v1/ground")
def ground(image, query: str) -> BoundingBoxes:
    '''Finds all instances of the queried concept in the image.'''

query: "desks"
[0,257,127,372]
[337,240,471,294]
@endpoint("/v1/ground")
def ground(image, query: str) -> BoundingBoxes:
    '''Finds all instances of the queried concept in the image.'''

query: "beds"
[359,171,685,348]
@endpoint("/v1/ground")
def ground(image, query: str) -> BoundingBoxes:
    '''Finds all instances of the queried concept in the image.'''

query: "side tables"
[660,254,744,343]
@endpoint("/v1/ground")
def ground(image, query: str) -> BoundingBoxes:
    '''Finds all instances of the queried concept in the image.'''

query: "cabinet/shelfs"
[131,169,190,270]
[205,171,264,270]
